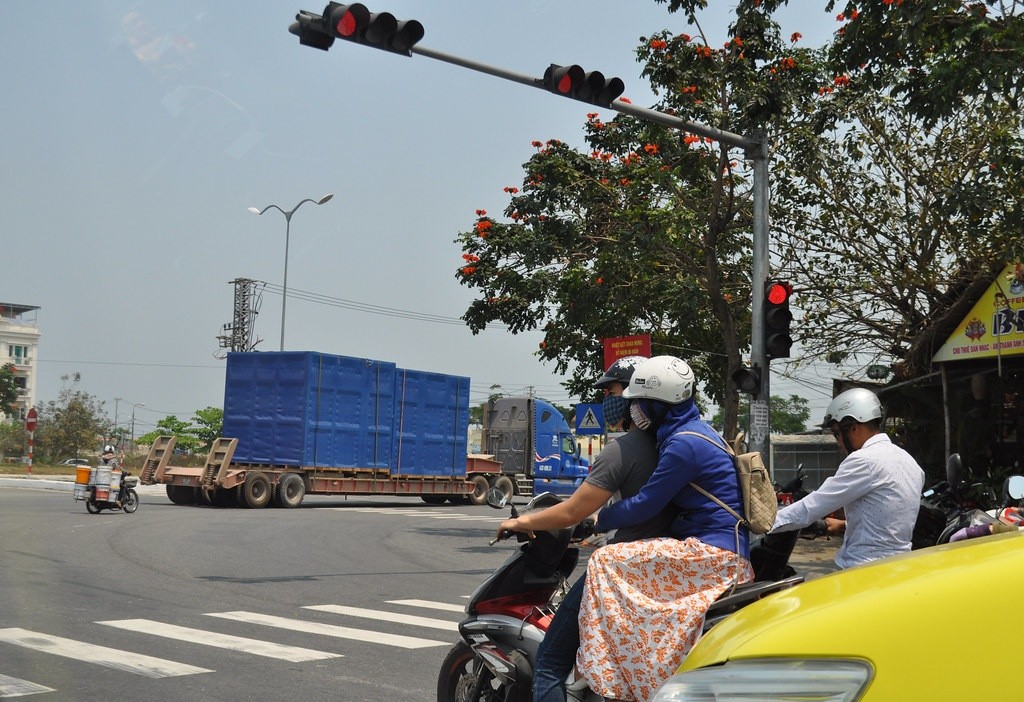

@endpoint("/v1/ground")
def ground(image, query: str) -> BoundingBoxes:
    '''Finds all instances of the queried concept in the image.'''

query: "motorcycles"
[83,470,140,515]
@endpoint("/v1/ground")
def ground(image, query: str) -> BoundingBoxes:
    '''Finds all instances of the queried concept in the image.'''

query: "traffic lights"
[731,366,764,396]
[543,61,626,110]
[322,1,426,58]
[762,278,794,361]
[287,9,335,51]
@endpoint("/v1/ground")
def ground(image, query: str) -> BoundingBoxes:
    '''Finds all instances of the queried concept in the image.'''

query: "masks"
[629,400,652,432]
[603,396,630,427]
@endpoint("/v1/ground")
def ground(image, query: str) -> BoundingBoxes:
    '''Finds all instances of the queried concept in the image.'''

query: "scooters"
[747,451,1024,584]
[433,488,808,702]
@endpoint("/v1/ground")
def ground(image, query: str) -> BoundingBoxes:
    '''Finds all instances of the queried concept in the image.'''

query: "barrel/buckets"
[73,465,122,503]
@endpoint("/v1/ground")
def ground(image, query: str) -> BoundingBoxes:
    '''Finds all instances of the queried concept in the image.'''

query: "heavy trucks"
[138,349,593,509]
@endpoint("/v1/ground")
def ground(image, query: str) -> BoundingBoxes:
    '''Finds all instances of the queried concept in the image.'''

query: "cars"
[646,529,1024,702]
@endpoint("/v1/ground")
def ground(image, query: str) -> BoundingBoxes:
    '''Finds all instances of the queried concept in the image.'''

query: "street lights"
[246,192,335,353]
[131,402,146,456]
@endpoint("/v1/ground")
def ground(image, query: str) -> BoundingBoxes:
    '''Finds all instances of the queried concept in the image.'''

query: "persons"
[498,355,678,702]
[100,444,127,508]
[957,396,995,475]
[767,387,925,570]
[566,356,754,702]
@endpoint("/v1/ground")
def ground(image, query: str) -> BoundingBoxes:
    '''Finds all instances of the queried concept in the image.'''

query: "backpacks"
[673,422,778,534]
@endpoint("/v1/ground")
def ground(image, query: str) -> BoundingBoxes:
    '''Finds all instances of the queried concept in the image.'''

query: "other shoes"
[115,501,122,510]
[563,664,589,690]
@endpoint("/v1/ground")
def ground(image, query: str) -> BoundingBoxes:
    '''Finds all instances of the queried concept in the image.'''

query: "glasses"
[834,423,850,441]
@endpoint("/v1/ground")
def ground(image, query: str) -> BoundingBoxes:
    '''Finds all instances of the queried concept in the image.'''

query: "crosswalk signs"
[575,402,606,435]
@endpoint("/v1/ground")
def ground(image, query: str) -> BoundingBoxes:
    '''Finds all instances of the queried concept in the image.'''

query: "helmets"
[621,355,695,405]
[592,356,648,389]
[105,445,115,452]
[824,388,885,425]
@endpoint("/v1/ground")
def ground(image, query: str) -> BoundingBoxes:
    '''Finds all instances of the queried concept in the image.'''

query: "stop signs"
[26,408,37,432]
